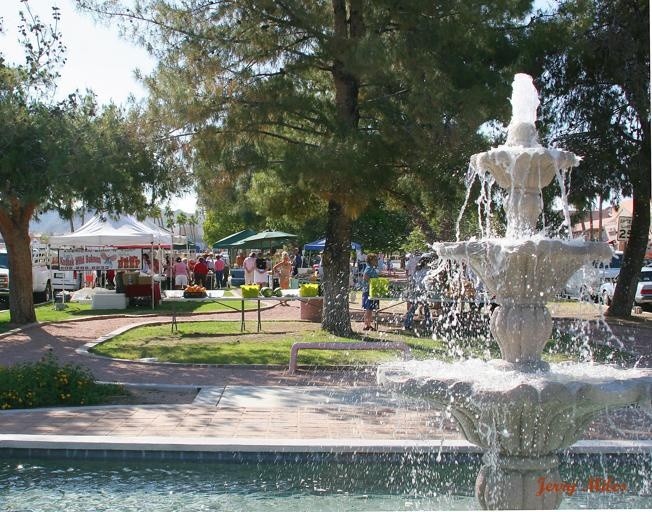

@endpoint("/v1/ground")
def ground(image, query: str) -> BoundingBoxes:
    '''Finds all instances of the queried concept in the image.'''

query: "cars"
[1,248,53,301]
[599,266,652,307]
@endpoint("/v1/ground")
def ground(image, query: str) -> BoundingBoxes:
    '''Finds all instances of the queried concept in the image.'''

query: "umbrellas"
[230,227,299,278]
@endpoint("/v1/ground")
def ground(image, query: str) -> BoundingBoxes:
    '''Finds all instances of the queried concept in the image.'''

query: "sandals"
[363,325,377,331]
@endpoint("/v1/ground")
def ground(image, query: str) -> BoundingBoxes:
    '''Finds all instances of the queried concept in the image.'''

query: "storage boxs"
[123,272,139,285]
[369,277,390,297]
[240,284,260,298]
[138,277,152,285]
[300,283,319,296]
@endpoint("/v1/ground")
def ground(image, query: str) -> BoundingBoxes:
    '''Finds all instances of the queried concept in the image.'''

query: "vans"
[558,251,624,303]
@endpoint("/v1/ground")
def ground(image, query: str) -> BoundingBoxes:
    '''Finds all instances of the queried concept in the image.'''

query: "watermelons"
[261,287,273,297]
[275,289,282,297]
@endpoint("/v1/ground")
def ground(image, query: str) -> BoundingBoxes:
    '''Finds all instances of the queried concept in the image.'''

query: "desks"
[369,296,494,331]
[125,282,161,309]
[162,295,296,333]
[190,272,213,289]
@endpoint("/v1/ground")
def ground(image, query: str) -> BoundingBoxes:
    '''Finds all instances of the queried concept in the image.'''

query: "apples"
[184,284,205,293]
